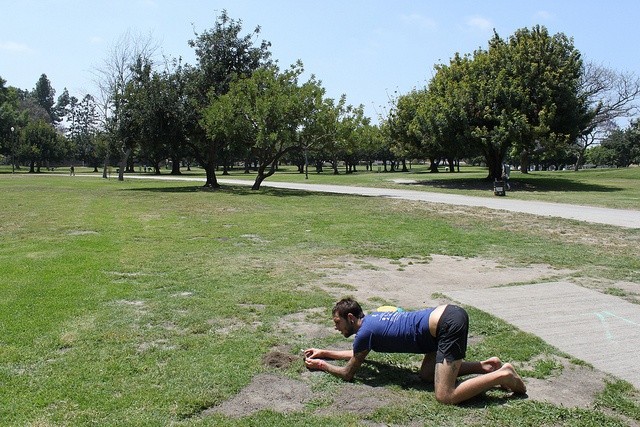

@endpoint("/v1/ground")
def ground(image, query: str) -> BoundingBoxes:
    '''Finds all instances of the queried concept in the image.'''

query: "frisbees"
[376,306,397,313]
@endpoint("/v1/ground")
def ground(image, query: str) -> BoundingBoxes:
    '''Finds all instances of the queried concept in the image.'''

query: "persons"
[304,297,527,405]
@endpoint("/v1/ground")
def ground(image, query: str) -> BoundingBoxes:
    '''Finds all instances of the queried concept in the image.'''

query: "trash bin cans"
[494,181,505,196]
